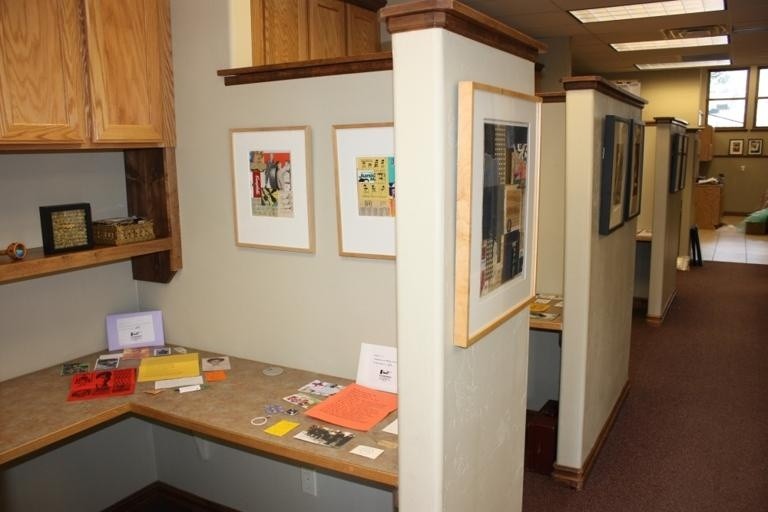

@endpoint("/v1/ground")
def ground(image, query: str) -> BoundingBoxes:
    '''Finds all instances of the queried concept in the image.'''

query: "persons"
[306,422,355,447]
[90,371,110,396]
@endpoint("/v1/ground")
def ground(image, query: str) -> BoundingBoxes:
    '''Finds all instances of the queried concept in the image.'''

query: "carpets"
[521,258,766,511]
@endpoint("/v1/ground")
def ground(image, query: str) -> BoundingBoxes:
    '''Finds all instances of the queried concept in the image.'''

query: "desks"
[528,291,563,335]
[0,346,397,492]
[636,227,653,243]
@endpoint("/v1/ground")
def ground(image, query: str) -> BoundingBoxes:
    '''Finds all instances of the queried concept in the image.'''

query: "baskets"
[93,223,155,245]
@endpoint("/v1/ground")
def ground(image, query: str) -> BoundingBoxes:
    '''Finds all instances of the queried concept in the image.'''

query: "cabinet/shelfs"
[693,181,724,229]
[697,124,714,162]
[249,0,349,70]
[345,0,387,59]
[2,1,182,284]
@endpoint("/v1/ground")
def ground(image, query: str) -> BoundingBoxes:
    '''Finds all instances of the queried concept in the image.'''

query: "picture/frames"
[36,202,93,255]
[331,119,396,263]
[728,138,763,157]
[600,114,645,237]
[225,124,317,255]
[106,309,167,356]
[454,81,543,348]
[670,134,687,194]
